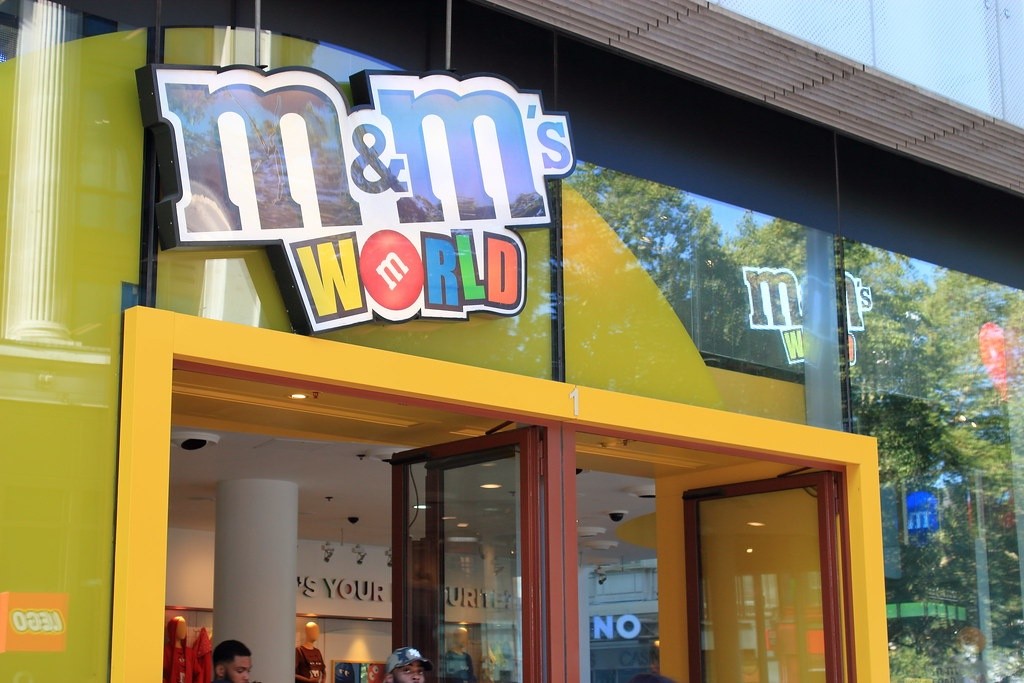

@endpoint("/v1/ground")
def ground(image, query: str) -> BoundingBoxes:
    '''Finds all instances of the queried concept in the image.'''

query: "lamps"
[352,544,366,565]
[594,568,607,583]
[322,541,333,562]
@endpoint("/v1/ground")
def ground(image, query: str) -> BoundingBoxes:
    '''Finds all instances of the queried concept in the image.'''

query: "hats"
[383,646,433,675]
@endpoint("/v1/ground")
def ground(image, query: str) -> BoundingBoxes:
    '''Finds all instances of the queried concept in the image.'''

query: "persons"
[445,627,477,683]
[165,616,194,683]
[295,622,327,683]
[212,639,253,683]
[384,648,425,683]
